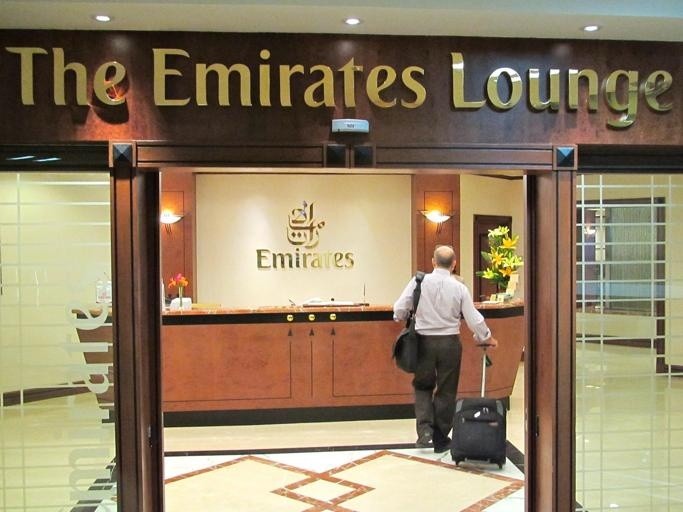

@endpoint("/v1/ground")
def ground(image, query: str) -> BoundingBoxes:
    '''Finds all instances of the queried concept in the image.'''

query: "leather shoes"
[416,430,452,453]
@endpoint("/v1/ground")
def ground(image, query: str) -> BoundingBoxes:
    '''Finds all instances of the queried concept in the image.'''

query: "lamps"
[160,215,183,224]
[419,210,457,223]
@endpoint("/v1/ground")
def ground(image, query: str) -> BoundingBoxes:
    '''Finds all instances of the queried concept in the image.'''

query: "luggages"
[451,343,506,470]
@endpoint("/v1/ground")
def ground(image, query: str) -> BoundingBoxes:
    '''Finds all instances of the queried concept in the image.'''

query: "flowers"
[168,273,188,288]
[475,226,524,289]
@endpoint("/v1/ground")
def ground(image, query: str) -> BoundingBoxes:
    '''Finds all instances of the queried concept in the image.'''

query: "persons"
[392,245,497,454]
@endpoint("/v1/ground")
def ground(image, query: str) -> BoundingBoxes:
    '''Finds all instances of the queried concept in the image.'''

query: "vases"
[179,286,183,308]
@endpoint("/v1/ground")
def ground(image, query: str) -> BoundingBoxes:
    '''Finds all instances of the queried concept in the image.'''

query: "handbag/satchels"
[392,326,419,373]
